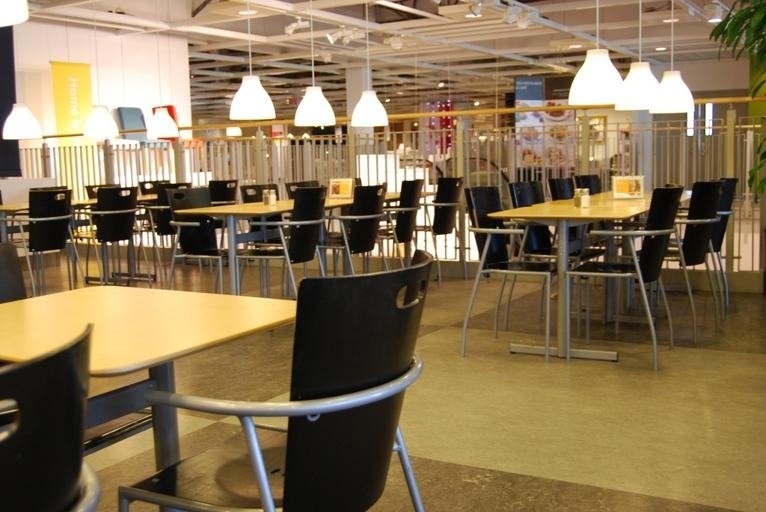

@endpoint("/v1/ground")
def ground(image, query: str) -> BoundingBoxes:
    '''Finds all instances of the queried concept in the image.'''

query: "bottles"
[262,189,278,208]
[572,188,590,208]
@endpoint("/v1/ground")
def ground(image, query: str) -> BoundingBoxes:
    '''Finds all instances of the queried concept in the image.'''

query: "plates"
[515,99,573,168]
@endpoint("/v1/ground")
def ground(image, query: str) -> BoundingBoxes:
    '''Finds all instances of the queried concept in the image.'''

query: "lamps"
[227,0,390,129]
[566,0,695,114]
[0,17,46,140]
[80,1,179,140]
